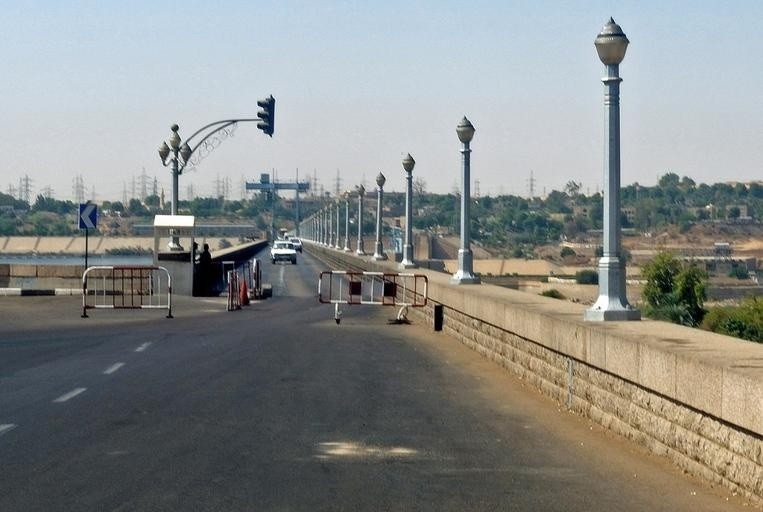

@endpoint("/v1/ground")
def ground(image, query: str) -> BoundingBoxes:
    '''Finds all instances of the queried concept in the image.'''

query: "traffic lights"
[254,93,275,137]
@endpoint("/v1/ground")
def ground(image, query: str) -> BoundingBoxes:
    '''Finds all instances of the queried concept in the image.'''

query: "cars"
[269,227,303,265]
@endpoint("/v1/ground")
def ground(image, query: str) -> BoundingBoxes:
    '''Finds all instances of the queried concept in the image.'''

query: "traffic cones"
[237,278,251,306]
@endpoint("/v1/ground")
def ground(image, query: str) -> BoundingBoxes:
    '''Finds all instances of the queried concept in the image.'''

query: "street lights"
[155,122,194,256]
[299,171,387,262]
[395,150,419,270]
[579,11,643,325]
[450,111,482,287]
